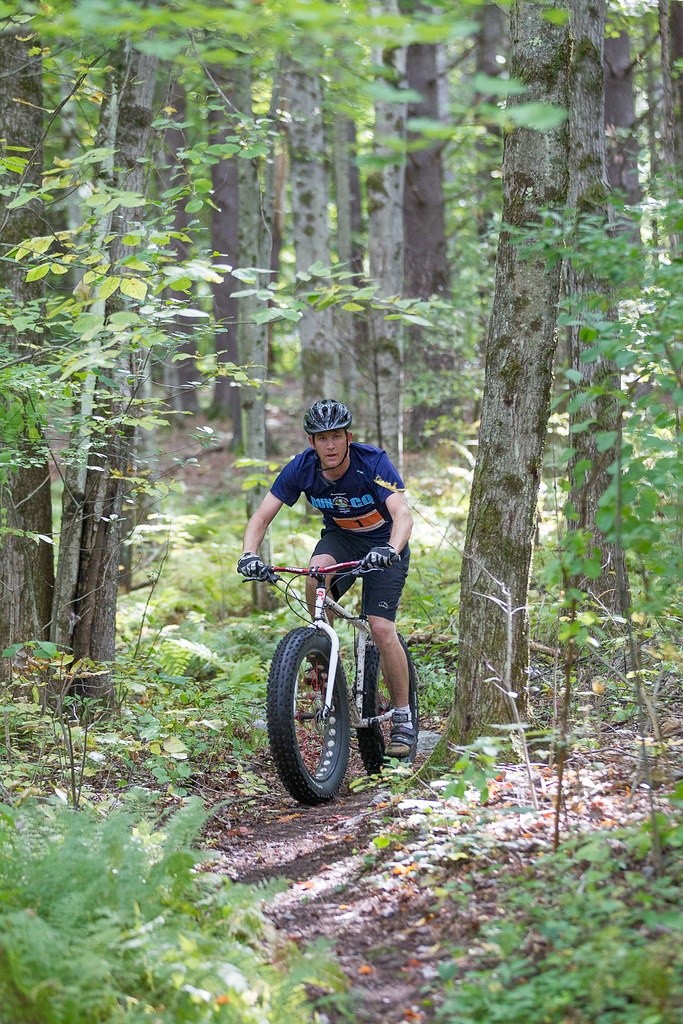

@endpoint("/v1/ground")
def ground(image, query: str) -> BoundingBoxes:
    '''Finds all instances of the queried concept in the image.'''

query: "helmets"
[303,399,351,434]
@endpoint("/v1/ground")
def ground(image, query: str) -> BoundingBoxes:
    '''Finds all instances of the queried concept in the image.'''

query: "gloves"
[237,552,272,578]
[362,543,402,569]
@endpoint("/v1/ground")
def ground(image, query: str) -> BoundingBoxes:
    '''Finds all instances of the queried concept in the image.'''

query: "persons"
[237,398,417,757]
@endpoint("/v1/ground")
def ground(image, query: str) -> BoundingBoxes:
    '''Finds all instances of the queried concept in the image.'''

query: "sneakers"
[304,667,318,684]
[384,712,416,756]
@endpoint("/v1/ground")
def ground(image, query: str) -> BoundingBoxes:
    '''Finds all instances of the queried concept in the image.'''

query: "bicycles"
[236,555,419,803]
